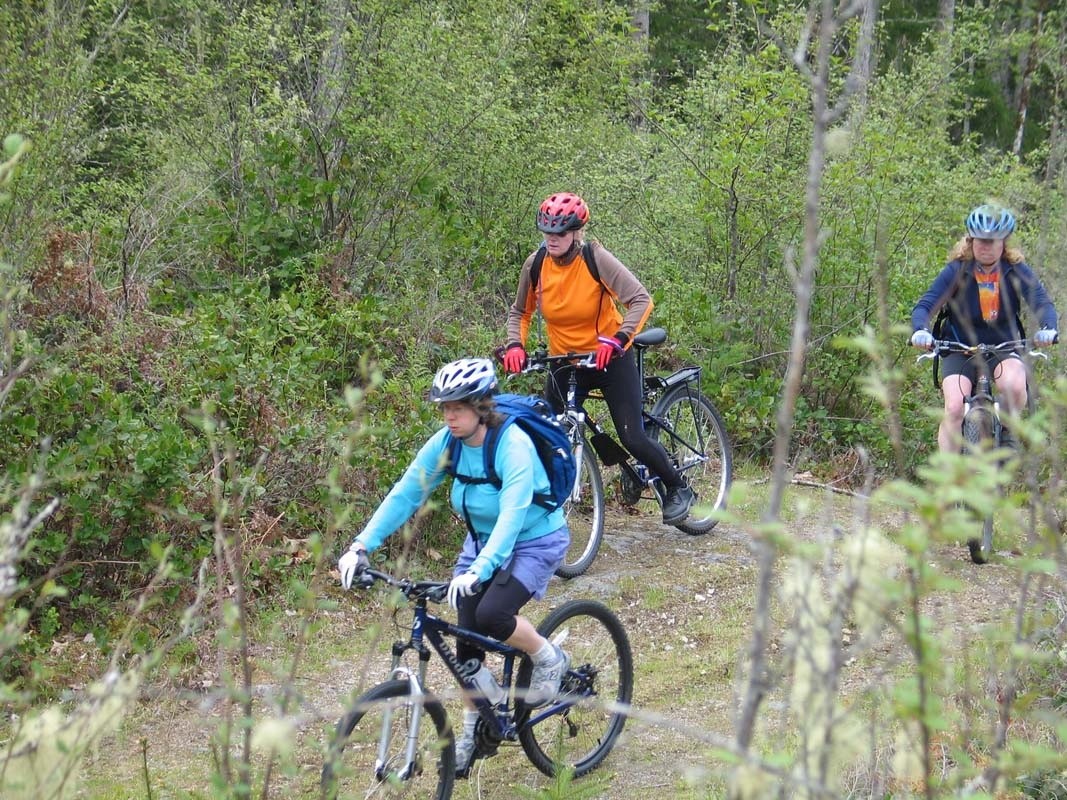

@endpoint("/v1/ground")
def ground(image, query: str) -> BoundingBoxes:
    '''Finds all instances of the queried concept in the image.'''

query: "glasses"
[544,230,574,237]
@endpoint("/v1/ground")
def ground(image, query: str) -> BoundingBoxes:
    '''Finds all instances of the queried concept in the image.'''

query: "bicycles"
[317,560,633,800]
[490,327,737,580]
[903,335,1063,561]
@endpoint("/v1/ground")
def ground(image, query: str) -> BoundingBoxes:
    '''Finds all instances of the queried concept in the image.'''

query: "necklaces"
[463,424,482,441]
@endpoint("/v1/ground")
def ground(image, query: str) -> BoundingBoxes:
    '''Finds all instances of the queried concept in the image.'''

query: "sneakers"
[435,718,479,780]
[662,482,697,525]
[524,643,572,710]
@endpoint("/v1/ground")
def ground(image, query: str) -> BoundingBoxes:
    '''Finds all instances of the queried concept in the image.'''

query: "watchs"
[349,544,367,553]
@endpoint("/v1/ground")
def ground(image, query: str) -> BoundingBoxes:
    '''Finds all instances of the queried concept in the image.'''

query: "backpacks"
[446,394,578,511]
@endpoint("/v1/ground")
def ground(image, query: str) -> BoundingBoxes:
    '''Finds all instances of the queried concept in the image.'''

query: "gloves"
[446,574,480,612]
[1033,324,1059,347]
[337,544,372,592]
[594,333,626,371]
[503,342,527,373]
[910,329,935,351]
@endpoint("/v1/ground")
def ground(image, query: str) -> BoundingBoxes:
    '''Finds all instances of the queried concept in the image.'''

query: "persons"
[910,205,1058,508]
[337,359,572,765]
[503,192,698,524]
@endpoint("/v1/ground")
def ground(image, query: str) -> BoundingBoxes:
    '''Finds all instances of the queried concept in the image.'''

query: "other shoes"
[1001,426,1018,452]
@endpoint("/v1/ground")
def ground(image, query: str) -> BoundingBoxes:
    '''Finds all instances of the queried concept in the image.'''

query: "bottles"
[465,658,505,705]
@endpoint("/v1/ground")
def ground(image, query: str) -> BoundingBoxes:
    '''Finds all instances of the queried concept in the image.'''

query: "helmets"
[429,358,499,403]
[966,204,1016,241]
[536,192,589,234]
